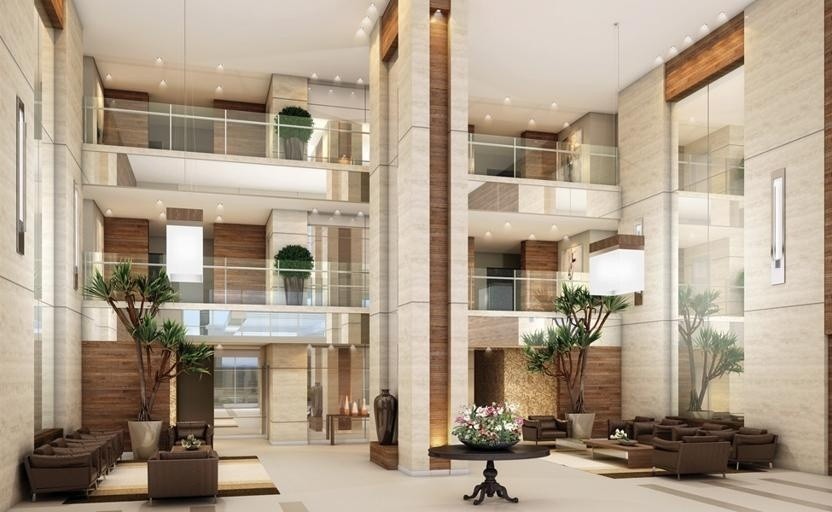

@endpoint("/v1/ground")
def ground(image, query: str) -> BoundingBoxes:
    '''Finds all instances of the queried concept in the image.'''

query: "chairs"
[522,415,778,480]
[23,421,218,506]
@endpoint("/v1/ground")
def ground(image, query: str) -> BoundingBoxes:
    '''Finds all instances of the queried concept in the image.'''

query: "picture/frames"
[560,242,583,297]
[560,127,583,183]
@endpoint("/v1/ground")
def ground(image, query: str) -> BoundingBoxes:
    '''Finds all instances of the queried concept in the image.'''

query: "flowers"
[452,403,524,442]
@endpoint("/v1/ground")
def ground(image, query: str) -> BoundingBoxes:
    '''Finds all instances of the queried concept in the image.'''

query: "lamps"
[589,234,645,296]
[165,207,204,284]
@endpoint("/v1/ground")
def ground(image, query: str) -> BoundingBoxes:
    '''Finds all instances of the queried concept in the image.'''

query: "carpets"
[62,455,281,504]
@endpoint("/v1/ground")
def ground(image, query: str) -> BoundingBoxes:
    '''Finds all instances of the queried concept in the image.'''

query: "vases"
[461,440,520,450]
[344,395,368,416]
[374,389,398,445]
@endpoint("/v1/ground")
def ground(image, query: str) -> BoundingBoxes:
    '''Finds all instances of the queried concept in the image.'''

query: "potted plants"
[79,257,215,461]
[521,282,633,441]
[274,106,315,160]
[678,283,745,419]
[274,244,315,305]
